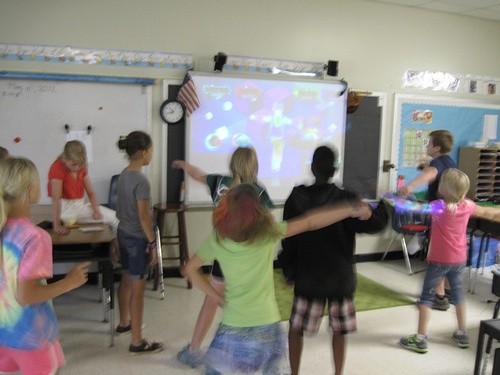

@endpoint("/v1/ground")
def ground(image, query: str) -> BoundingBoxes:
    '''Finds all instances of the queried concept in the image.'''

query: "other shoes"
[129,340,164,355]
[116,319,131,335]
[177,345,203,368]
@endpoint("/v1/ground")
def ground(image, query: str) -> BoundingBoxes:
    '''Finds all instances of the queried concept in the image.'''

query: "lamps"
[214,52,227,72]
[324,60,339,76]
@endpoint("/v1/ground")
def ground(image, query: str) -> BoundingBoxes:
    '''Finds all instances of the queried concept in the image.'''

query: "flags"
[176,73,200,116]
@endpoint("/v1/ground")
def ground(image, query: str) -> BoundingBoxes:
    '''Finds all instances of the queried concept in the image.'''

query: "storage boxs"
[472,238,500,268]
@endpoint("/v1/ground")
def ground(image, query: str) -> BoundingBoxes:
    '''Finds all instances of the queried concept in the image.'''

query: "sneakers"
[451,329,470,347]
[400,335,428,353]
[431,294,450,310]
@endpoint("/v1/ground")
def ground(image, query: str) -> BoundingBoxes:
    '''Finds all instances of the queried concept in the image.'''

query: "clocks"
[159,98,186,124]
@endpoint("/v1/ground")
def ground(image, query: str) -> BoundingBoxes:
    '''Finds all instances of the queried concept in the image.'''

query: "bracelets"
[150,239,156,250]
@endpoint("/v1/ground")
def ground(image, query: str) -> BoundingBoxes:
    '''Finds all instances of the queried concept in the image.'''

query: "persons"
[182,183,371,375]
[172,146,272,369]
[115,131,164,355]
[47,141,122,266]
[400,129,456,310]
[278,146,394,375]
[392,168,500,353]
[0,156,91,375]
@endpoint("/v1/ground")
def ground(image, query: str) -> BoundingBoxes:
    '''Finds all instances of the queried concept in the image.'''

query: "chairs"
[382,201,430,276]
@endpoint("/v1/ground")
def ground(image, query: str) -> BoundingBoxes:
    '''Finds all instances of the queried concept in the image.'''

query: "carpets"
[202,269,416,321]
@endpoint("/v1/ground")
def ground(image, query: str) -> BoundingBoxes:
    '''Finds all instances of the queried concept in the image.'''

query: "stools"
[109,175,164,300]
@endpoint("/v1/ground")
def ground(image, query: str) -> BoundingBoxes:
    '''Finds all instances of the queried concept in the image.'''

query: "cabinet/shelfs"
[457,146,500,203]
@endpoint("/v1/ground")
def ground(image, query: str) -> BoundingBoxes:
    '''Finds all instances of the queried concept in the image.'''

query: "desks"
[153,201,193,291]
[31,204,115,347]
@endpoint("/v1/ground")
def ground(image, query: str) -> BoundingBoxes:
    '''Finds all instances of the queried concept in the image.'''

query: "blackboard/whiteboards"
[390,93,500,195]
[160,79,387,204]
[0,71,153,205]
